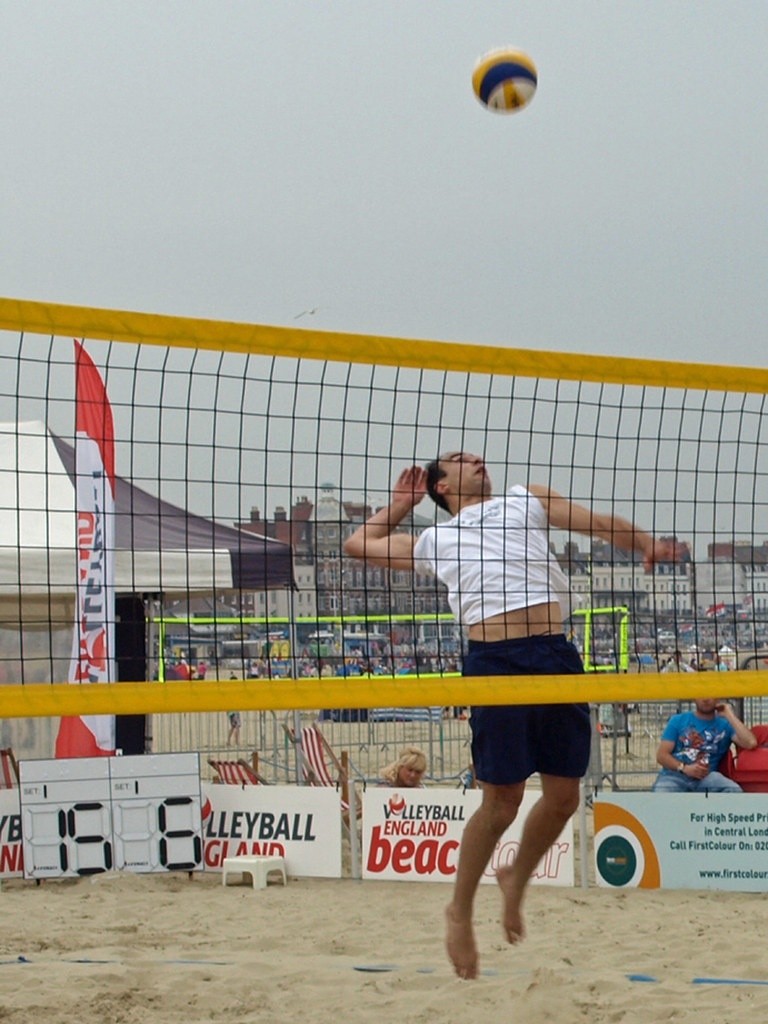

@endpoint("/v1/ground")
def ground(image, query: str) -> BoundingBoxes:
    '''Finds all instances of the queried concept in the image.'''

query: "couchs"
[720,724,768,794]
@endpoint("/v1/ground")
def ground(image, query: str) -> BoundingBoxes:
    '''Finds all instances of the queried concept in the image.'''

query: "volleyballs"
[471,46,539,113]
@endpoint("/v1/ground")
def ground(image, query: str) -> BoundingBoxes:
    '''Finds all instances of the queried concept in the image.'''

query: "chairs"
[207,759,272,786]
[281,721,364,854]
[0,747,20,789]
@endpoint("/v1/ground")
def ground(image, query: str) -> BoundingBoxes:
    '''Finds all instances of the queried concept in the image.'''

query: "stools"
[221,854,289,891]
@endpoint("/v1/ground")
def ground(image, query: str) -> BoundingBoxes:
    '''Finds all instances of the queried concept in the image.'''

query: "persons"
[659,650,695,674]
[343,450,690,981]
[227,676,242,747]
[651,699,758,793]
[378,748,423,792]
[713,654,727,670]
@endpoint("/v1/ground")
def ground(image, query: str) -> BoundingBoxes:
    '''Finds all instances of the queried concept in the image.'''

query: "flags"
[54,338,115,757]
